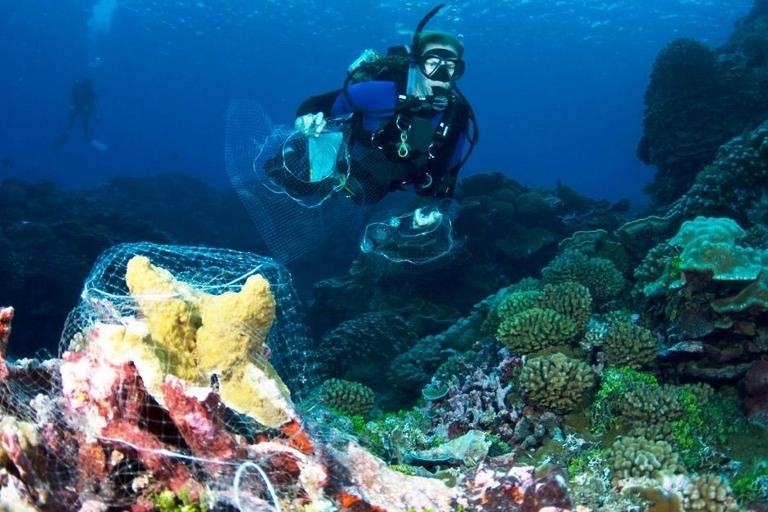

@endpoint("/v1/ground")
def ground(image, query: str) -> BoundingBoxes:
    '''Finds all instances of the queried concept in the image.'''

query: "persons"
[261,32,480,266]
